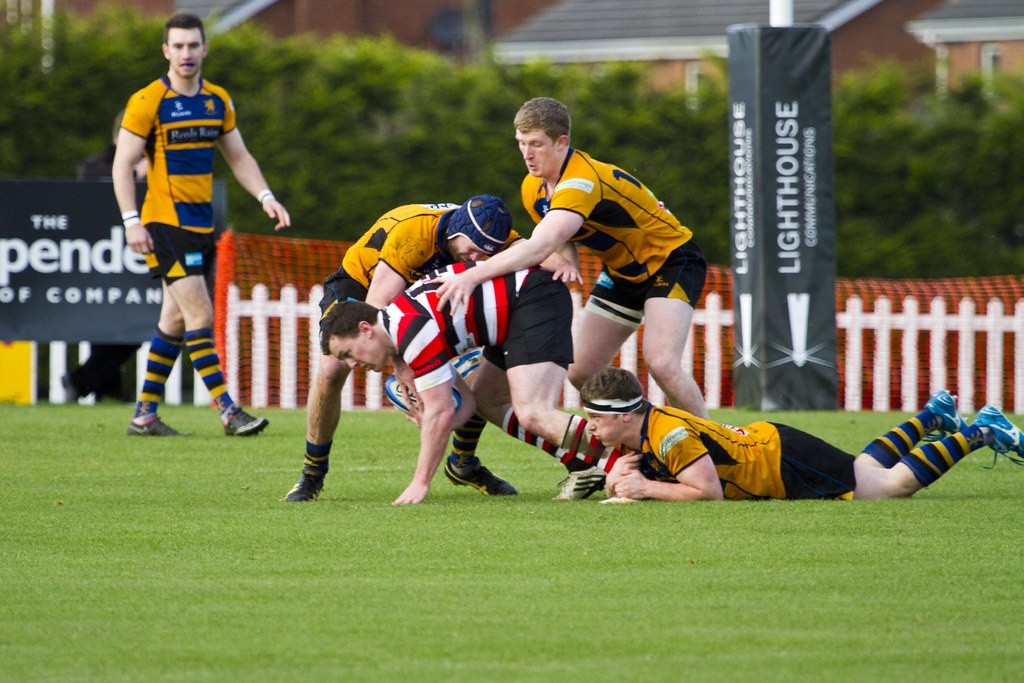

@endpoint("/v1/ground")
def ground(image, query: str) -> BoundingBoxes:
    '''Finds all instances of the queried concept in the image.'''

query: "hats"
[444,194,512,255]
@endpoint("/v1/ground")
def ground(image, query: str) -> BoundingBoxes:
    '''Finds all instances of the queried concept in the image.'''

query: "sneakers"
[127,415,179,437]
[445,455,519,497]
[222,407,269,437]
[552,465,607,500]
[285,465,329,501]
[924,388,1024,465]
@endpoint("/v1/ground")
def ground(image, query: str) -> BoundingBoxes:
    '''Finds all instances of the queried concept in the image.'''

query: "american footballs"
[383,371,462,417]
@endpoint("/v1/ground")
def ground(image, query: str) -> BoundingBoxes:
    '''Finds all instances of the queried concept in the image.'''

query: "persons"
[579,367,1024,499]
[286,99,709,503]
[112,13,292,436]
[59,108,149,404]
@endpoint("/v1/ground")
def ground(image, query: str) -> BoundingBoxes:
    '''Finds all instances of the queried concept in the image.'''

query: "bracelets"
[121,211,141,229]
[257,189,275,203]
